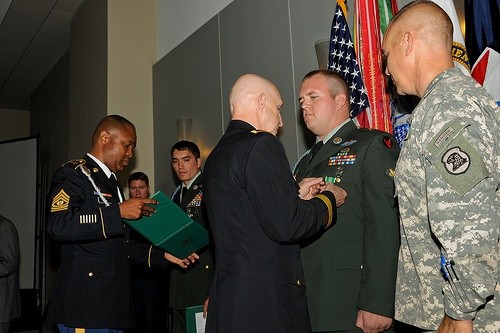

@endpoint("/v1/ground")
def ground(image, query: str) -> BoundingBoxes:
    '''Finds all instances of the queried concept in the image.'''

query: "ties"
[307,140,323,163]
[110,173,118,197]
[182,186,188,200]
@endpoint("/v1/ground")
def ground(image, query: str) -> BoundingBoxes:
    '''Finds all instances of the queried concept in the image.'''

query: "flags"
[328,0,500,145]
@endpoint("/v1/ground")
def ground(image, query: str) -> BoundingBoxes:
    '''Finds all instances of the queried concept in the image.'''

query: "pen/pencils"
[439,255,460,282]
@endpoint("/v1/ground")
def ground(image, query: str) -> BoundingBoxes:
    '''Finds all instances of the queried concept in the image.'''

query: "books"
[121,190,209,260]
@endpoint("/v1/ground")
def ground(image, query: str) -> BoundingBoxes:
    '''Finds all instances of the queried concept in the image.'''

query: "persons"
[381,0,500,333]
[202,73,348,333]
[46,114,200,333]
[292,70,402,333]
[0,216,21,333]
[128,171,150,199]
[164,140,215,333]
